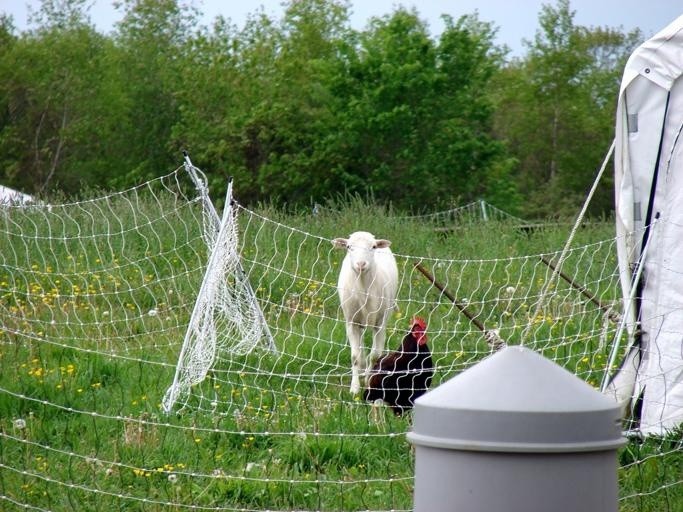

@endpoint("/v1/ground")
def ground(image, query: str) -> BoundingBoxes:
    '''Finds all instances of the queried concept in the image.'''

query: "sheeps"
[330,229,400,393]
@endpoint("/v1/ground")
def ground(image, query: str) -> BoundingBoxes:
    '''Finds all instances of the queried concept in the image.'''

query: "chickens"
[364,316,434,424]
